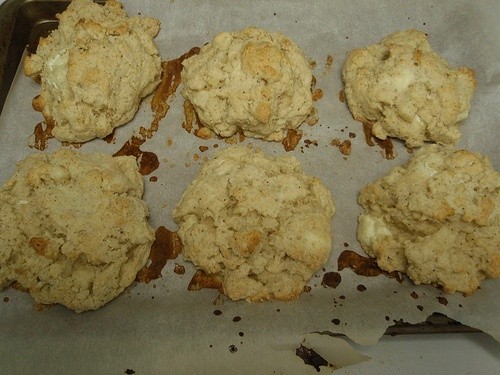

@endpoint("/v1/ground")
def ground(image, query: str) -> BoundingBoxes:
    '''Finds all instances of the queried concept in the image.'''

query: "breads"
[0,1,500,312]
